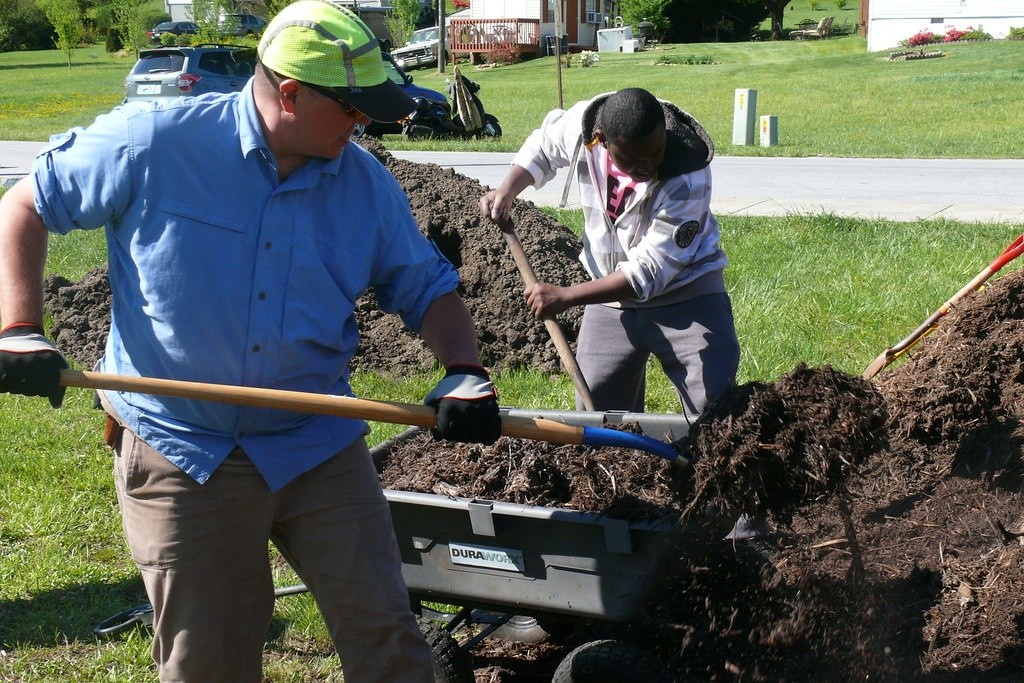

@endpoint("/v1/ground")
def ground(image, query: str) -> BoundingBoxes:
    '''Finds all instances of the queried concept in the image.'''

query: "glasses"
[297,80,362,117]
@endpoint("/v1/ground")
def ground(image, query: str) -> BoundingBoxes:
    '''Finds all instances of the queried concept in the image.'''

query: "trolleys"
[93,405,703,683]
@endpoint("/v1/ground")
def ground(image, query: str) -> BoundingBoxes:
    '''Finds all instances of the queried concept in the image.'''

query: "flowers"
[899,26,992,48]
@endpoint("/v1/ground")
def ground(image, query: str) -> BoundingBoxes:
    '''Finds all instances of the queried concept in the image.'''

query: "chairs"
[823,16,835,36]
[788,17,829,40]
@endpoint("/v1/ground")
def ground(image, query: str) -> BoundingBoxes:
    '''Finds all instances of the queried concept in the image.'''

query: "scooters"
[402,66,502,143]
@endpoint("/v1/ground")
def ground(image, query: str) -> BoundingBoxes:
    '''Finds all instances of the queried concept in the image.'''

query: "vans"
[217,13,266,37]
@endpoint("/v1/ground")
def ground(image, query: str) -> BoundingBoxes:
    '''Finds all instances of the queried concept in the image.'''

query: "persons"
[478,87,740,415]
[0,0,502,683]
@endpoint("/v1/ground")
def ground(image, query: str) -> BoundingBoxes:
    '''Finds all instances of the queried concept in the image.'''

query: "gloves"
[424,365,502,445]
[0,323,69,408]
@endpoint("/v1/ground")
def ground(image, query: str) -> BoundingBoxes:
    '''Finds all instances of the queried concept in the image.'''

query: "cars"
[146,21,203,45]
[350,51,449,137]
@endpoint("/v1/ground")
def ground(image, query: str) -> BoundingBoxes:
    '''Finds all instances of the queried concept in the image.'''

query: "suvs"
[390,25,471,70]
[124,43,255,98]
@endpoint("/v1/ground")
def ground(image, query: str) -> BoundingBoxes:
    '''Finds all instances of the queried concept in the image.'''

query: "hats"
[256,0,418,123]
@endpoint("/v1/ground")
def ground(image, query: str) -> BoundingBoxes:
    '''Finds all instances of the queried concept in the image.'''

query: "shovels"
[63,372,777,488]
[862,232,1024,382]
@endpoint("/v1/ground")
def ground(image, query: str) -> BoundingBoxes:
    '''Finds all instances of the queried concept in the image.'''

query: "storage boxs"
[370,404,705,618]
